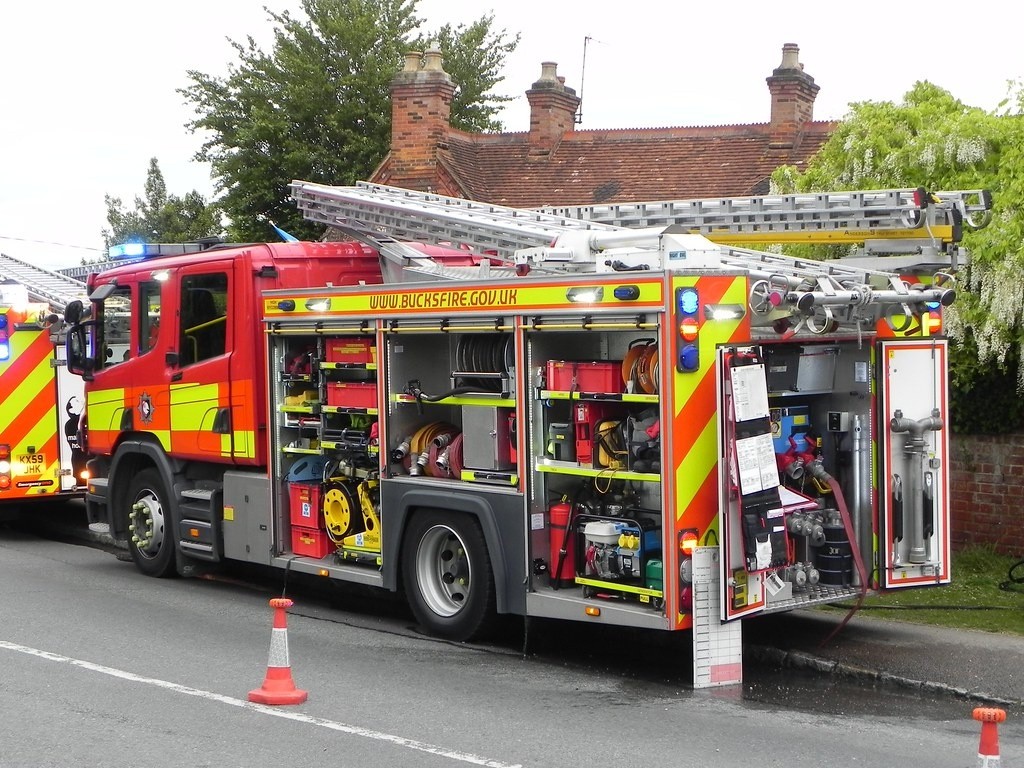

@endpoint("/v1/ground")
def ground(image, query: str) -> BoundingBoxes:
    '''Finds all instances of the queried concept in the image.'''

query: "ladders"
[289,177,994,332]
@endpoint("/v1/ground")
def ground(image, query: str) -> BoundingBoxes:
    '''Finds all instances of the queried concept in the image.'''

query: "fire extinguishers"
[547,488,576,588]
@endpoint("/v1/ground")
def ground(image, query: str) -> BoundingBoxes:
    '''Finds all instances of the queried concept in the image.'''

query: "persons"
[64,396,94,486]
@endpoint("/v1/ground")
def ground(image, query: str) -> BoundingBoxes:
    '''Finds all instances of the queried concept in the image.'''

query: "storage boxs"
[546,360,627,396]
[549,422,577,462]
[461,404,517,470]
[288,481,338,559]
[325,337,379,409]
[763,344,839,392]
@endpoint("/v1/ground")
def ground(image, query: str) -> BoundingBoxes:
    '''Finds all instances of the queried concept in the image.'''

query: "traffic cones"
[249,598,309,707]
[972,707,1006,768]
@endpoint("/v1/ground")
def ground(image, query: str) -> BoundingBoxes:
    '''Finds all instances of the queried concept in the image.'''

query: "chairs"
[179,289,226,367]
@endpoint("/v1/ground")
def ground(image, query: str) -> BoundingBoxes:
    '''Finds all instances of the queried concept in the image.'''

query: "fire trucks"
[0,285,160,504]
[64,179,994,644]
